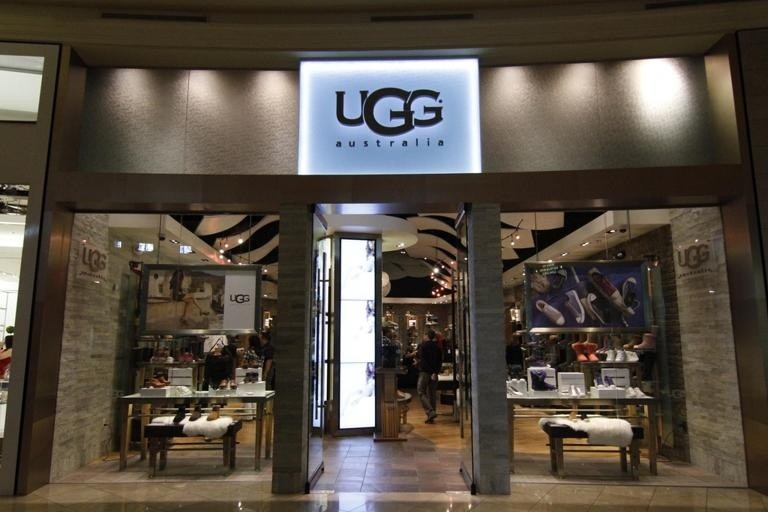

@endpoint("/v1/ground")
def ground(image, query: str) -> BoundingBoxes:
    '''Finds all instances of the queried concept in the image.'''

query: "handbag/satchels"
[206,354,232,371]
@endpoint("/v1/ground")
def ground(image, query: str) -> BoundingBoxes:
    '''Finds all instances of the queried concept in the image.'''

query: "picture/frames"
[522,260,650,333]
[141,264,261,335]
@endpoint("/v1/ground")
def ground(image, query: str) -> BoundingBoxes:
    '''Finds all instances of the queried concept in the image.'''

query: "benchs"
[144,418,242,477]
[542,423,644,479]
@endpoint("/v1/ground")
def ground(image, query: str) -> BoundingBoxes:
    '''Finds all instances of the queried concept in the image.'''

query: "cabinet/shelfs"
[135,363,205,390]
[527,361,643,417]
[377,368,412,441]
[234,366,263,420]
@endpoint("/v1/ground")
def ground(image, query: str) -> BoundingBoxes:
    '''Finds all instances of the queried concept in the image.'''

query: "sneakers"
[626,387,645,397]
[245,372,258,383]
[566,272,640,327]
[596,376,615,389]
[536,300,565,325]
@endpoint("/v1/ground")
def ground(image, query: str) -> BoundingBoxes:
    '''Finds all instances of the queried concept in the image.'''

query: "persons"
[170,271,210,325]
[363,300,375,334]
[197,340,236,406]
[240,335,264,422]
[415,329,442,425]
[0,335,15,378]
[262,334,276,393]
[356,238,375,273]
[404,347,418,385]
[363,362,375,400]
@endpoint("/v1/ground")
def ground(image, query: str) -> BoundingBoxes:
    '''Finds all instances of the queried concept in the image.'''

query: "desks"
[506,393,659,476]
[116,389,275,471]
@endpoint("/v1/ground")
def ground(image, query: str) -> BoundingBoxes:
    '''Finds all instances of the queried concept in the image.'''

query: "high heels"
[146,377,169,388]
[200,312,209,316]
[179,319,189,326]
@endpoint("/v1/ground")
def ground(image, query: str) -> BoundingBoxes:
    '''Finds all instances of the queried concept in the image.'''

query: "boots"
[241,351,259,368]
[525,333,655,362]
[173,406,185,422]
[207,405,219,420]
[531,370,556,390]
[189,404,201,420]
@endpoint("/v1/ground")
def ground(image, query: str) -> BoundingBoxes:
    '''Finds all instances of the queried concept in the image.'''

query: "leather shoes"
[569,385,585,396]
[219,380,237,389]
[424,413,437,423]
[509,386,523,396]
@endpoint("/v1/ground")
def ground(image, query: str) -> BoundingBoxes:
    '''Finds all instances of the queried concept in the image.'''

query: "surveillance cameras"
[619,228,627,233]
[159,235,167,241]
